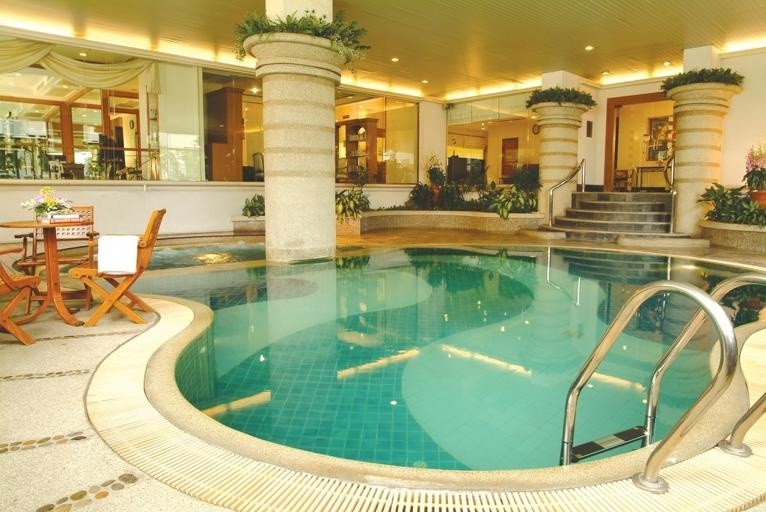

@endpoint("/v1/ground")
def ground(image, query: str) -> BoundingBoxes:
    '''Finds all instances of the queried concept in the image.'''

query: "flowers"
[745,147,766,190]
[23,187,71,212]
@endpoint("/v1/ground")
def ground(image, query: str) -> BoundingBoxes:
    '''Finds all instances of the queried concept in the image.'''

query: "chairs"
[252,153,264,181]
[1,205,165,345]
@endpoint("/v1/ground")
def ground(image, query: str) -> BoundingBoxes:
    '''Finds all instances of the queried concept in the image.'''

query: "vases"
[747,190,766,211]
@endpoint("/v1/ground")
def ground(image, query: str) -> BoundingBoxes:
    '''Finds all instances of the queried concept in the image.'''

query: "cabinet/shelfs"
[336,118,377,183]
